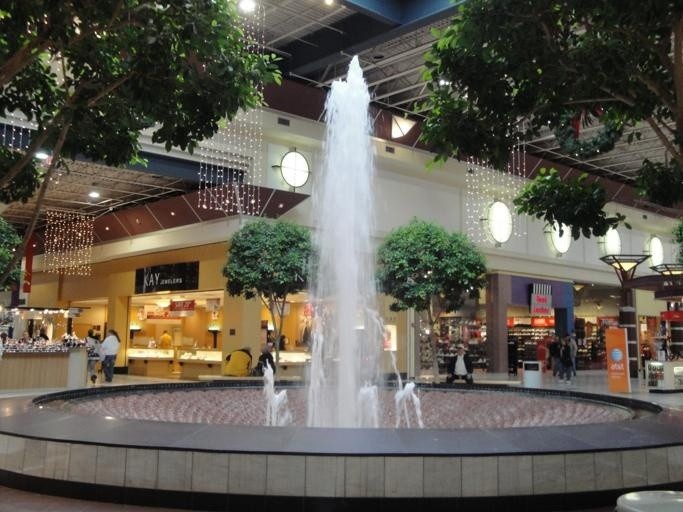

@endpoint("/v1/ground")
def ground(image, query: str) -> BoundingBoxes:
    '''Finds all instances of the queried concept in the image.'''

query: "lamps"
[272,147,312,193]
[479,198,512,248]
[642,234,664,267]
[543,219,572,256]
[391,114,416,139]
[596,227,621,260]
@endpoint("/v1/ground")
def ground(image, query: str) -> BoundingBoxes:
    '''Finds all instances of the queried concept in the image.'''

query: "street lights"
[649,262,683,291]
[599,254,652,379]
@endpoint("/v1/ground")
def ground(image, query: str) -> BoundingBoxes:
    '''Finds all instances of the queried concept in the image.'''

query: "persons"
[445,343,473,386]
[19,331,33,345]
[99,329,121,382]
[221,345,252,378]
[549,334,578,385]
[83,329,99,384]
[158,330,172,350]
[250,346,276,377]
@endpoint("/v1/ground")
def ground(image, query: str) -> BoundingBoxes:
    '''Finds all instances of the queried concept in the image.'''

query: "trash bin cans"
[523,361,542,388]
[657,349,665,363]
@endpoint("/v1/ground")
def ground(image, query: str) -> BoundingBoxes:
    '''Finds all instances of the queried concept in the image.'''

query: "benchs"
[454,379,520,387]
[198,375,301,382]
